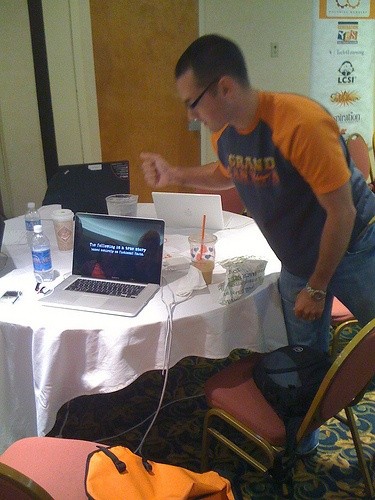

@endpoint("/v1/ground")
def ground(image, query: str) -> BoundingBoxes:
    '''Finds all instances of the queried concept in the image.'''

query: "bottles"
[24,202,41,252]
[29,224,54,283]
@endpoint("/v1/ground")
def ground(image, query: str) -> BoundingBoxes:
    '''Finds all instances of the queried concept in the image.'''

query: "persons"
[139,33,375,455]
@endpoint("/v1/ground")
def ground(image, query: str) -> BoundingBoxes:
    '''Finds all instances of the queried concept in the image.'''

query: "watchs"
[306,283,327,300]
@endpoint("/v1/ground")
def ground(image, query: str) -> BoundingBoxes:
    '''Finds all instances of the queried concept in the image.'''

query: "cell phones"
[1,291,22,305]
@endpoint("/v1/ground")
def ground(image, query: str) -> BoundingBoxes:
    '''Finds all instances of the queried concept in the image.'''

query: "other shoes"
[295,427,320,456]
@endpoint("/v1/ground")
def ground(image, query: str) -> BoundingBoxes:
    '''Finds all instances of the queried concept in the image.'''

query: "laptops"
[39,212,165,318]
[152,191,233,230]
[45,160,130,216]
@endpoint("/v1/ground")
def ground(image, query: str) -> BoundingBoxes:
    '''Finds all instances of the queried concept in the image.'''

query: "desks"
[1,201,283,446]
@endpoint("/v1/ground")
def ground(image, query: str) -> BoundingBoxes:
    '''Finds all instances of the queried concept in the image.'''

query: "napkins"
[159,255,207,297]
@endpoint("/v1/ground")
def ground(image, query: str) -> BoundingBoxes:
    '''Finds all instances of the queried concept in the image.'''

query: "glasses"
[185,77,217,112]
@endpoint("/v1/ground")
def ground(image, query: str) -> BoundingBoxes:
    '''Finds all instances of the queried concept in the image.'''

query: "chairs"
[200,317,375,500]
[328,294,360,363]
[179,162,248,217]
[345,132,375,194]
[0,437,112,500]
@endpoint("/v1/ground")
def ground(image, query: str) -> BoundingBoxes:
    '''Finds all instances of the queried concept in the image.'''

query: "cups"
[106,193,139,218]
[52,209,75,251]
[189,234,217,284]
